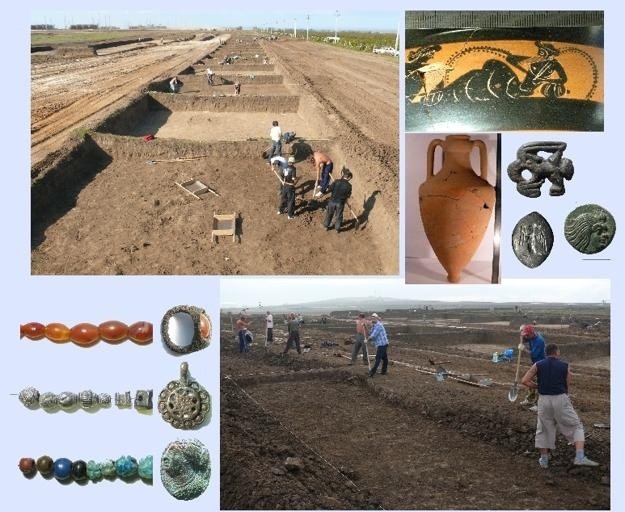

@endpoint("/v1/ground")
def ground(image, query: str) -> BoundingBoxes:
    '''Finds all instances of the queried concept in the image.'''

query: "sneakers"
[277,210,285,214]
[539,457,549,468]
[316,191,322,196]
[520,398,538,412]
[288,215,297,219]
[574,457,599,467]
[324,223,343,233]
[317,185,323,188]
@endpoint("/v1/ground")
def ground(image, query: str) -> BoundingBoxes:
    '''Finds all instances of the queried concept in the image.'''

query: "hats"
[521,325,535,336]
[288,157,296,163]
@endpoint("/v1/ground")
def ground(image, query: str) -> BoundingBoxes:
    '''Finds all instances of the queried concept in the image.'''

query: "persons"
[522,343,600,469]
[267,120,284,165]
[263,311,274,341]
[236,316,254,354]
[517,324,546,411]
[234,81,241,95]
[279,312,302,355]
[276,156,299,220]
[318,172,354,233]
[206,68,214,86]
[346,313,369,366]
[270,156,288,195]
[364,313,390,378]
[168,77,178,93]
[565,211,610,255]
[305,151,333,198]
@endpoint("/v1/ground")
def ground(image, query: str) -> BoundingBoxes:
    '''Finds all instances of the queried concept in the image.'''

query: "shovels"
[362,325,373,384]
[307,179,318,209]
[262,145,273,157]
[437,367,489,380]
[508,336,524,403]
[478,378,527,388]
[328,173,361,231]
[429,358,451,366]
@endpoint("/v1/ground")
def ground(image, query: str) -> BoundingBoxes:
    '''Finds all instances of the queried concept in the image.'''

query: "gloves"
[518,344,526,351]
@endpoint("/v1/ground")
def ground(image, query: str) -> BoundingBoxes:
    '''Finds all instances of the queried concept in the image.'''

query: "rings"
[161,303,213,354]
